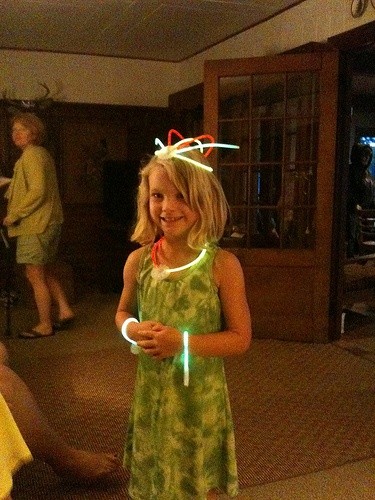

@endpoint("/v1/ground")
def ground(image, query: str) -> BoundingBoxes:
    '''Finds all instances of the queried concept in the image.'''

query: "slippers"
[51,315,78,330]
[18,326,55,339]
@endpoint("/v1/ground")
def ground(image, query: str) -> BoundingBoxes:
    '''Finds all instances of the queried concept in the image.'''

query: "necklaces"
[151,235,207,282]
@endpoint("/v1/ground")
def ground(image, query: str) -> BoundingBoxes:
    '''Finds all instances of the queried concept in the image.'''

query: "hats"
[350,140,373,169]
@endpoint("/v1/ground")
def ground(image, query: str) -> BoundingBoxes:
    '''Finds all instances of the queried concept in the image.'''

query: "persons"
[116,145,252,500]
[0,112,75,339]
[1,341,124,500]
[345,141,375,259]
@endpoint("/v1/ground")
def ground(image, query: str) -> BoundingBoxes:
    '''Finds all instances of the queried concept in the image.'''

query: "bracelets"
[183,330,190,386]
[121,318,140,346]
